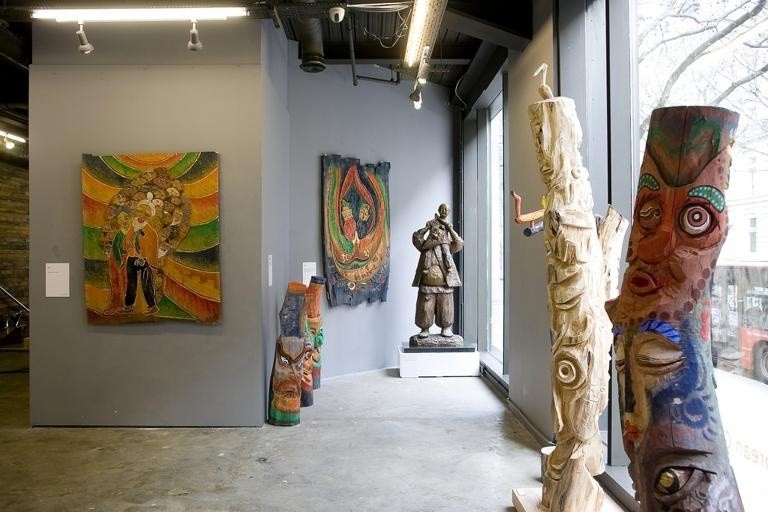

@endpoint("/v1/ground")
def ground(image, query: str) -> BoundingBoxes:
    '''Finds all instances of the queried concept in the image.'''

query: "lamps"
[28,0,250,21]
[409,46,431,109]
[187,20,203,51]
[1,122,26,150]
[75,21,94,54]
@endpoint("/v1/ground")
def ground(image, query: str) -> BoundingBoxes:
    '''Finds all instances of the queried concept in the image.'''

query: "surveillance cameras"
[329,6,345,23]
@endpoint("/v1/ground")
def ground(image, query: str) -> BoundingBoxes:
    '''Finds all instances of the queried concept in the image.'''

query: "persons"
[421,204,455,274]
[341,204,357,242]
[406,216,464,338]
[355,204,371,238]
[98,166,193,316]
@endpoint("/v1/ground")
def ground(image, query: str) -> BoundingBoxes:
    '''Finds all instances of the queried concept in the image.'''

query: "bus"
[709,261,767,383]
